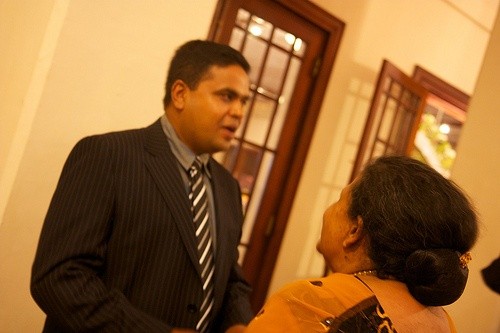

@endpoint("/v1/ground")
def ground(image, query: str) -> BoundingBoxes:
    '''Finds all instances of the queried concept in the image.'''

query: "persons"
[241,153,480,333]
[30,40,256,333]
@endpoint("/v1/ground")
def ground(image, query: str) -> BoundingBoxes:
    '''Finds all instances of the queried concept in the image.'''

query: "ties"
[187,158,216,333]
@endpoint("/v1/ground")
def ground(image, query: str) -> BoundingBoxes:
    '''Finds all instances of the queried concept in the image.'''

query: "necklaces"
[352,269,379,277]
